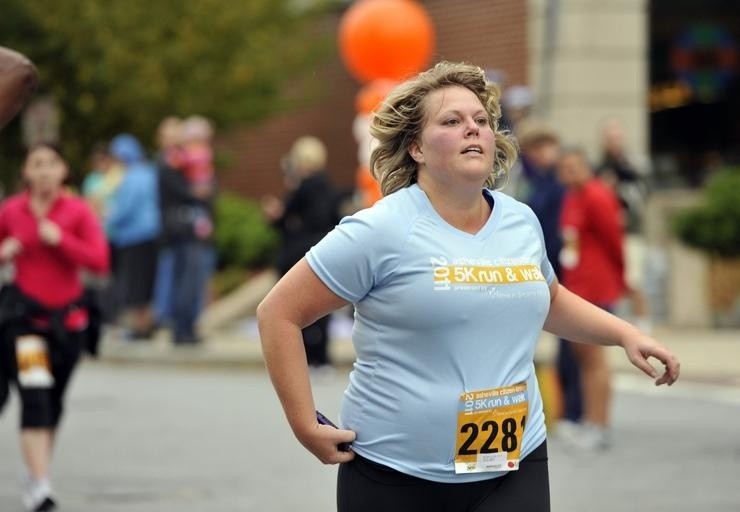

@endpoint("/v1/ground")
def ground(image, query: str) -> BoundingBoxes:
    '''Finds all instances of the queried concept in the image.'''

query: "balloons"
[337,0,436,208]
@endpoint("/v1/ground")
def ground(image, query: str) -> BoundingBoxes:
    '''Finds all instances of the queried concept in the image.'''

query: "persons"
[492,90,651,450]
[81,115,218,359]
[263,138,338,367]
[0,141,111,512]
[256,60,680,512]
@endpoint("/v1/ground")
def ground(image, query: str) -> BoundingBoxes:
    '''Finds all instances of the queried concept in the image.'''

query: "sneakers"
[21,483,58,512]
